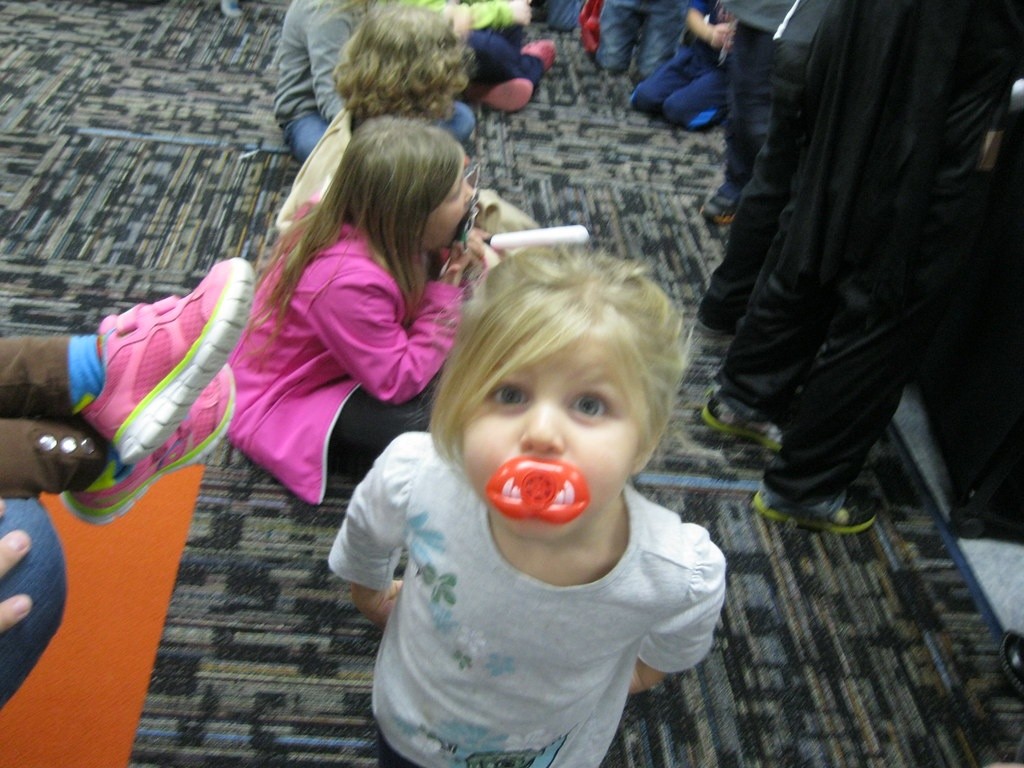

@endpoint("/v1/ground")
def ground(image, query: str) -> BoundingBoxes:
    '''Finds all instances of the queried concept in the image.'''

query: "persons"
[277,0,539,237]
[271,0,556,166]
[224,116,502,504]
[595,0,690,78]
[329,244,727,768]
[699,0,1024,534]
[630,0,738,133]
[0,255,254,634]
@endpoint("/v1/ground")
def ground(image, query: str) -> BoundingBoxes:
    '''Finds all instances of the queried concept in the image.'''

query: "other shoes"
[700,181,742,224]
[519,39,557,72]
[462,78,534,113]
[1000,632,1024,690]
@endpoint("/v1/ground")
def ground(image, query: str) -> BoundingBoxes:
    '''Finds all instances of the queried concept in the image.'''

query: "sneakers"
[753,480,878,533]
[701,383,783,453]
[57,363,236,524]
[80,258,255,465]
